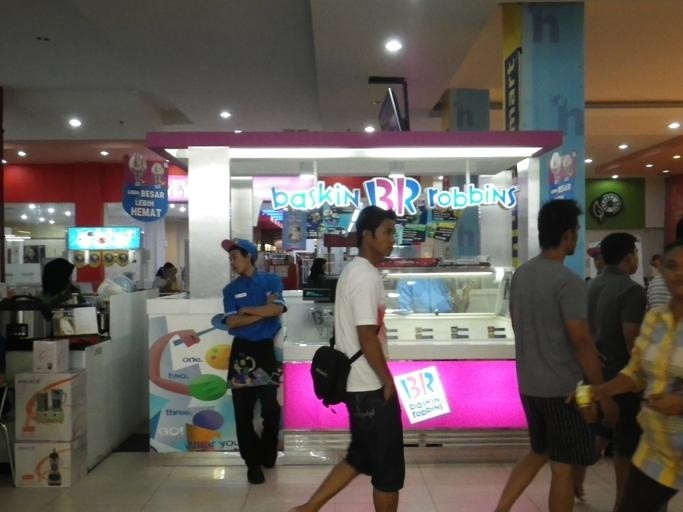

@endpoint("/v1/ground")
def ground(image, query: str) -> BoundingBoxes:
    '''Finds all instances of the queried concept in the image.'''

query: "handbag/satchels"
[309,345,352,415]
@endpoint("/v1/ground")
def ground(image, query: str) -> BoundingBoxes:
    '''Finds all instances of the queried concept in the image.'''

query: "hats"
[221,236,258,261]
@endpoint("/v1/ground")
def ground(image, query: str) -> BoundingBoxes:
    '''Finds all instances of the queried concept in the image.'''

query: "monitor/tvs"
[377,87,404,131]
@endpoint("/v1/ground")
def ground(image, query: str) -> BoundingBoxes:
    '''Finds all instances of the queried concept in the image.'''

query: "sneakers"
[246,445,278,484]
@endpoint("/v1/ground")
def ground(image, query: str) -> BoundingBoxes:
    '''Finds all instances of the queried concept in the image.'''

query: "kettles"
[35,389,66,424]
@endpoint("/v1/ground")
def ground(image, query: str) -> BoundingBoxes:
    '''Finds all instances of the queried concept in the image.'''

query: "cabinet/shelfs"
[280,263,533,463]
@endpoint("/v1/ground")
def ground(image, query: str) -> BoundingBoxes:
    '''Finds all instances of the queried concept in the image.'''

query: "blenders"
[47,448,62,487]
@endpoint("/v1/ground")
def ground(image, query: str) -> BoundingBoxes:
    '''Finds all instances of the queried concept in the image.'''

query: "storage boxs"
[32,338,69,373]
[14,367,87,441]
[13,436,88,490]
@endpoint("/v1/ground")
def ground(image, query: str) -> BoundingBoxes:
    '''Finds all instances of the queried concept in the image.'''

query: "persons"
[490,198,620,512]
[294,205,404,512]
[42,258,86,304]
[308,258,327,275]
[574,219,683,512]
[153,260,179,292]
[222,238,288,485]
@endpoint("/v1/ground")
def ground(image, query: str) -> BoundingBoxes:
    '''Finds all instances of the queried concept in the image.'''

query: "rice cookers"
[0,295,47,343]
